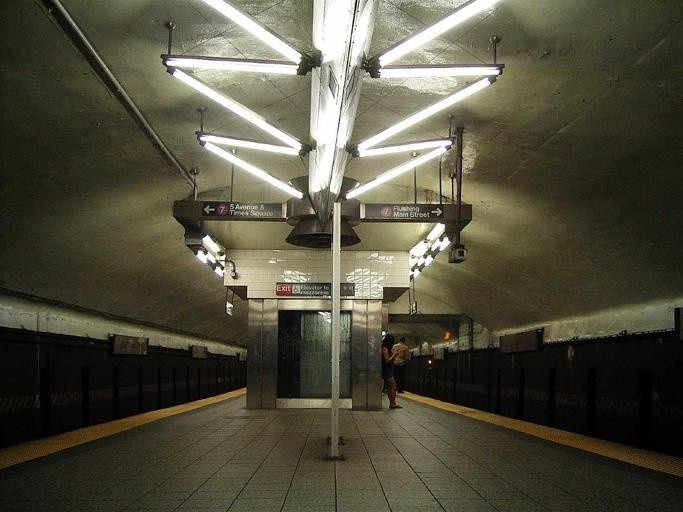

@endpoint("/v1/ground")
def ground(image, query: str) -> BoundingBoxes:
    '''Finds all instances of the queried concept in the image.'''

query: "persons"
[382,333,403,409]
[391,337,410,393]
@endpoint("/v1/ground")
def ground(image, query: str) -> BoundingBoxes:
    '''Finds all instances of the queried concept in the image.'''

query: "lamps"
[190,226,229,279]
[409,222,460,280]
[162,0,333,194]
[335,3,506,201]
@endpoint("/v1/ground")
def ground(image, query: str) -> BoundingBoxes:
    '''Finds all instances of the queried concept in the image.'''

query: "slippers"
[389,404,402,408]
